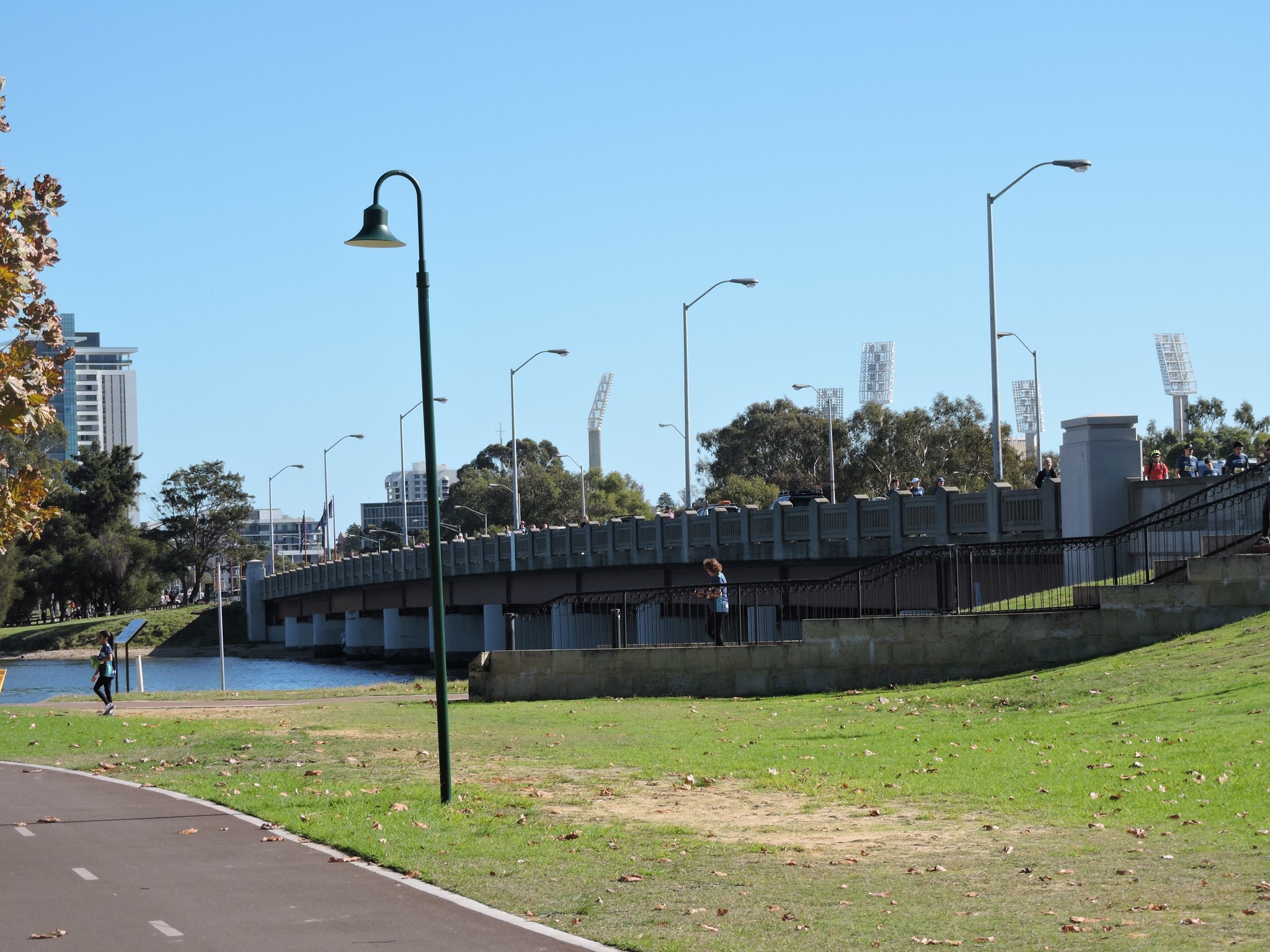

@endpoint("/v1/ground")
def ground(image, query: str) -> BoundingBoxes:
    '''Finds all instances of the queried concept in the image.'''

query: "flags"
[300,515,305,553]
[322,506,324,549]
[315,509,326,533]
[328,498,333,519]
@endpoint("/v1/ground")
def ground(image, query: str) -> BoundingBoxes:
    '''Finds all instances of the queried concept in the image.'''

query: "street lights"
[349,533,381,555]
[792,380,837,505]
[281,535,321,562]
[508,346,570,533]
[682,277,760,514]
[454,505,488,537]
[411,518,462,539]
[555,453,586,526]
[244,545,286,573]
[268,464,305,574]
[488,483,522,526]
[997,331,1044,476]
[984,150,1090,493]
[368,528,415,548]
[343,166,454,807]
[324,434,364,562]
[397,397,448,549]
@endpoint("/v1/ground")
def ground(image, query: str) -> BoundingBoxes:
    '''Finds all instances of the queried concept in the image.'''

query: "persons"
[1254,439,1270,547]
[910,478,924,497]
[931,478,946,496]
[1224,441,1249,475]
[1142,450,1169,480]
[91,630,116,714]
[1255,453,1265,464]
[159,586,188,610]
[696,559,729,646]
[1175,443,1199,478]
[906,482,913,490]
[1035,458,1057,489]
[886,479,901,498]
[40,602,110,624]
[1202,457,1219,476]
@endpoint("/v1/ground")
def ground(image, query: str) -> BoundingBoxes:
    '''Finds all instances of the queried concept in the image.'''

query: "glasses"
[1265,448,1270,451]
[1151,455,1160,458]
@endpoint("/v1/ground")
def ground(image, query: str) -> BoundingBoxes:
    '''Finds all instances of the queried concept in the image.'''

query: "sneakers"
[103,702,116,715]
[109,711,115,716]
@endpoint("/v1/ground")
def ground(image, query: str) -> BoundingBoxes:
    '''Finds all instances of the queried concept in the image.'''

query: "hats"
[912,478,921,483]
[1183,444,1193,450]
[936,477,946,482]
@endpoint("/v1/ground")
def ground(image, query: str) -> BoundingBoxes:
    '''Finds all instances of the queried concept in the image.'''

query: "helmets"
[1150,450,1161,456]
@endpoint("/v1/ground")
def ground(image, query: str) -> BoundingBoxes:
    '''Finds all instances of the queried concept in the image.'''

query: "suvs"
[694,499,742,516]
[1195,460,1230,476]
[768,488,828,511]
[229,589,242,602]
[213,590,231,604]
